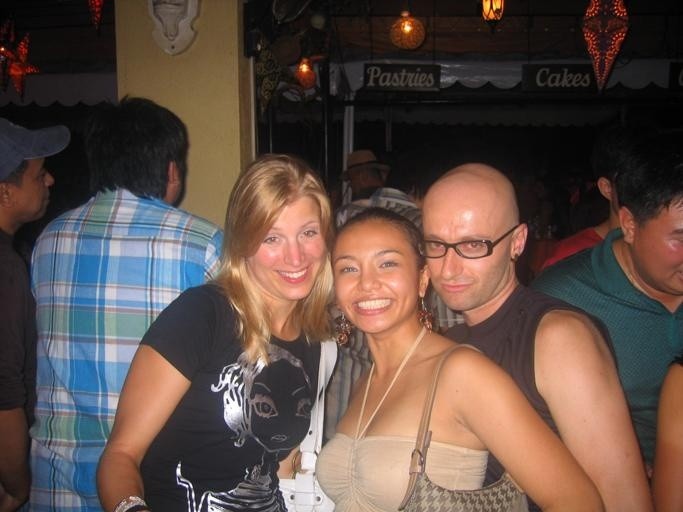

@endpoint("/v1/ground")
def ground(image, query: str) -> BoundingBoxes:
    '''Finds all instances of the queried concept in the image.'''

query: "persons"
[0,119,71,512]
[423,163,654,512]
[315,207,606,512]
[540,127,683,272]
[337,150,391,200]
[19,98,226,512]
[531,132,683,488]
[651,357,683,512]
[96,154,338,512]
[323,172,465,446]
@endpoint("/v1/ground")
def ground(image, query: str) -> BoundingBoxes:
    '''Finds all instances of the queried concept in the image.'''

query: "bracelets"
[114,495,149,512]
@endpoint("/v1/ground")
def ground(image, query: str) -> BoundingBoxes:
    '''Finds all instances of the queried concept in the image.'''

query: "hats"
[0,120,70,177]
[337,150,391,180]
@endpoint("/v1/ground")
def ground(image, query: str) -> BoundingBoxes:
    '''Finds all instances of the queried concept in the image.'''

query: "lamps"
[387,0,426,53]
[292,28,326,86]
[479,0,505,33]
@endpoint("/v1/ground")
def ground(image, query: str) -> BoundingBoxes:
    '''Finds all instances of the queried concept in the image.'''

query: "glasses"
[417,226,517,258]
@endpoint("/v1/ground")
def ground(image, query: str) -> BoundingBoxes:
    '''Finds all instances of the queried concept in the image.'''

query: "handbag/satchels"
[401,344,530,512]
[279,474,333,512]
